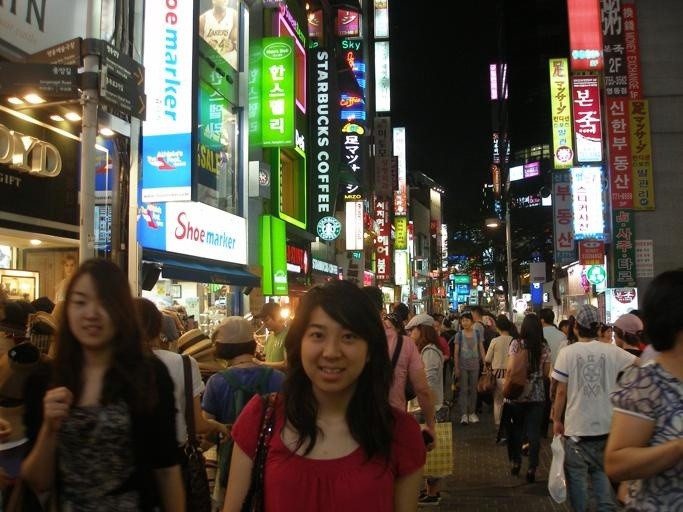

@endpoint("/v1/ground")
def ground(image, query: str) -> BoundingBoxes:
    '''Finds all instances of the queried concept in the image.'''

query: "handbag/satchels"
[412,405,448,424]
[177,445,214,512]
[241,480,263,512]
[476,374,496,394]
[4,472,62,512]
[548,432,568,504]
[501,350,528,398]
[415,421,454,478]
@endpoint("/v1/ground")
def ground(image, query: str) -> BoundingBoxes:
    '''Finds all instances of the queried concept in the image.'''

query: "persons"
[199,0,238,70]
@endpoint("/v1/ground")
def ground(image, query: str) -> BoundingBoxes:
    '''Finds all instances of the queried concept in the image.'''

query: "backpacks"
[216,368,274,487]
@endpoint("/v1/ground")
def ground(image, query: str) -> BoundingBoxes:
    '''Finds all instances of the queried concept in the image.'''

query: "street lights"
[484,201,513,323]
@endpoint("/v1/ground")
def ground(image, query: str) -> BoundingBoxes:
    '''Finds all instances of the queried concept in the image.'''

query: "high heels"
[510,462,538,487]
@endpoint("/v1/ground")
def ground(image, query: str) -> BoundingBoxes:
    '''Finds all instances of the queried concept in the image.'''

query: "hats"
[612,314,645,336]
[574,305,601,333]
[403,314,436,331]
[176,314,255,370]
[253,302,281,318]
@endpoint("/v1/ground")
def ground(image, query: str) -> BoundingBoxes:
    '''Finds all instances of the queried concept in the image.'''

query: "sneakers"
[460,413,480,426]
[415,488,442,506]
[494,434,510,445]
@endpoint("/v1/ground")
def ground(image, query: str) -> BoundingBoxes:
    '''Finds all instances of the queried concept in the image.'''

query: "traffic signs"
[99,38,145,93]
[0,61,77,99]
[97,68,146,123]
[14,38,82,76]
